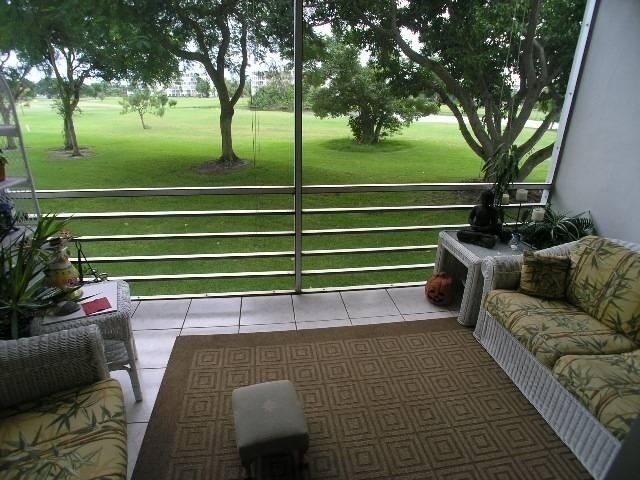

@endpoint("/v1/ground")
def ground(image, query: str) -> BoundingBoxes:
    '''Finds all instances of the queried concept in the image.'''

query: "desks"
[433,228,544,327]
[32,279,143,402]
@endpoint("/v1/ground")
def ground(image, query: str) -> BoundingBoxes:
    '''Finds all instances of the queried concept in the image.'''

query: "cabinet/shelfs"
[0,74,42,299]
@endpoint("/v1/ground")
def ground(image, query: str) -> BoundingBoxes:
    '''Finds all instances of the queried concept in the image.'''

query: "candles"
[501,188,546,221]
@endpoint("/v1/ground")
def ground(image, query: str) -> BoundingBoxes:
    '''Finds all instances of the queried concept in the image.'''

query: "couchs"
[0,323,129,480]
[473,235,640,480]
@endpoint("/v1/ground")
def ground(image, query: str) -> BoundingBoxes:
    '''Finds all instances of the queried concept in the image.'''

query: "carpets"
[130,317,594,480]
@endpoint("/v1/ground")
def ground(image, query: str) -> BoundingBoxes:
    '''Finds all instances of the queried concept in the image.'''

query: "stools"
[231,379,310,479]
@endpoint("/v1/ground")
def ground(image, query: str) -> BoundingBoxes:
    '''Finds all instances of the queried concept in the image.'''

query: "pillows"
[522,250,571,297]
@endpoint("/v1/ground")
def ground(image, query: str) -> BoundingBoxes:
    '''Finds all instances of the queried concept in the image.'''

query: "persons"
[457,190,499,249]
